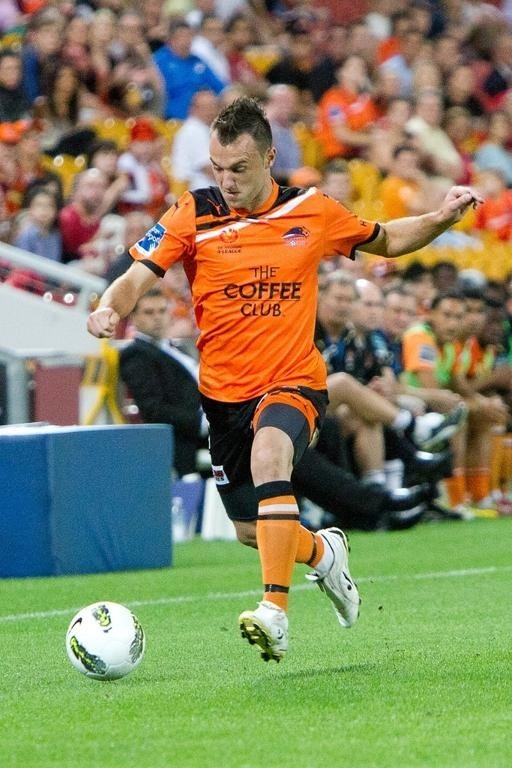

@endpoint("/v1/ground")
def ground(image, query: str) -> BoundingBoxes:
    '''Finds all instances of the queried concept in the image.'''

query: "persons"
[86,95,485,663]
[2,2,512,529]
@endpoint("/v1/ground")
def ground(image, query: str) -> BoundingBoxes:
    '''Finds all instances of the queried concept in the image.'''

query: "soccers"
[65,600,146,682]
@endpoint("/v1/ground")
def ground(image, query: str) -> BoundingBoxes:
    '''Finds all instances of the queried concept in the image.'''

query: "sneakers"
[405,407,468,451]
[238,600,289,664]
[304,527,362,629]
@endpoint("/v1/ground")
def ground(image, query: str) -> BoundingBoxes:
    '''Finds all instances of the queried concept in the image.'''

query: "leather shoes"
[382,484,429,530]
